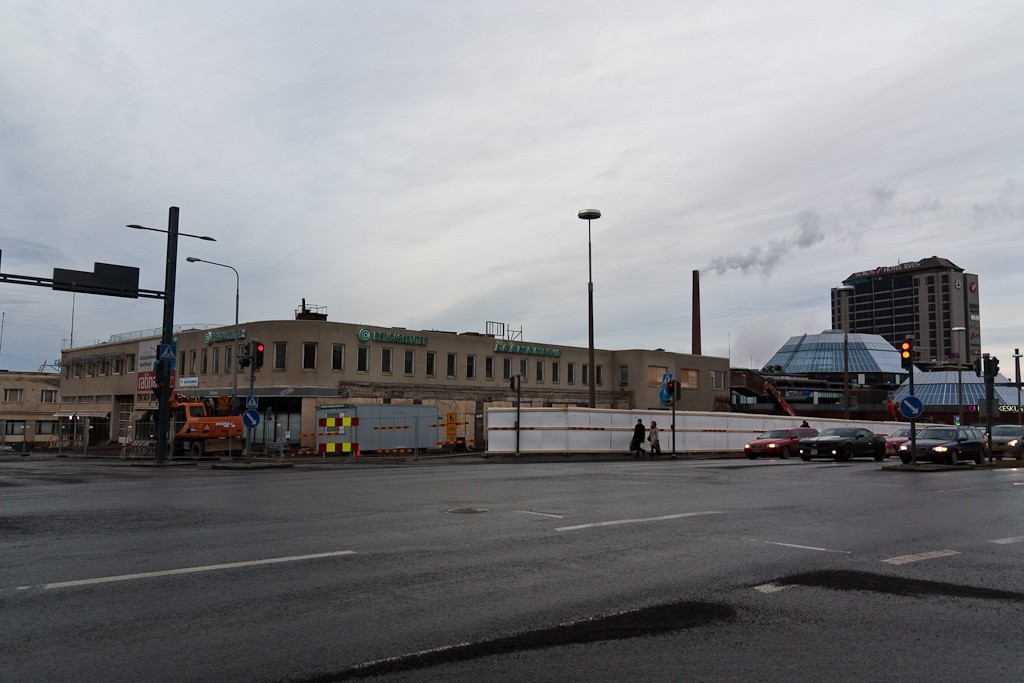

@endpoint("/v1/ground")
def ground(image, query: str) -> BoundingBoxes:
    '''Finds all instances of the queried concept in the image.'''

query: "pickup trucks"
[165,401,246,460]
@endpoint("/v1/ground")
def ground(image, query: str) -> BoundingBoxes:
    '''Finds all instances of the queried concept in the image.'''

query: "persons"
[648,421,661,456]
[800,420,810,428]
[631,418,646,458]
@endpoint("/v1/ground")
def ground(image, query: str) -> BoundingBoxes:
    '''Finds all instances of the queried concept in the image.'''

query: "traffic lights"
[240,357,250,367]
[253,341,264,368]
[667,381,674,393]
[974,358,981,377]
[992,358,1000,376]
[901,340,912,369]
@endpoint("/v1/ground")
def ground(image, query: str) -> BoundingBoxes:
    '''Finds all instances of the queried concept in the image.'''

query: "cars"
[798,425,888,462]
[884,426,925,458]
[897,424,986,466]
[743,427,820,460]
[984,424,1024,461]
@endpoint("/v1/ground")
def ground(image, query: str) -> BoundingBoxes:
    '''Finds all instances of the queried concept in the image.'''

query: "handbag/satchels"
[630,440,636,451]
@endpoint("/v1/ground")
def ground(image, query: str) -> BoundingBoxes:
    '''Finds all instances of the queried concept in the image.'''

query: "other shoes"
[635,454,639,457]
[643,450,645,455]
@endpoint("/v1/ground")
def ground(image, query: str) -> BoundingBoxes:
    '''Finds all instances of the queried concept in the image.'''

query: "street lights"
[577,207,602,409]
[125,206,217,469]
[185,256,240,416]
[952,326,967,371]
[836,284,855,419]
